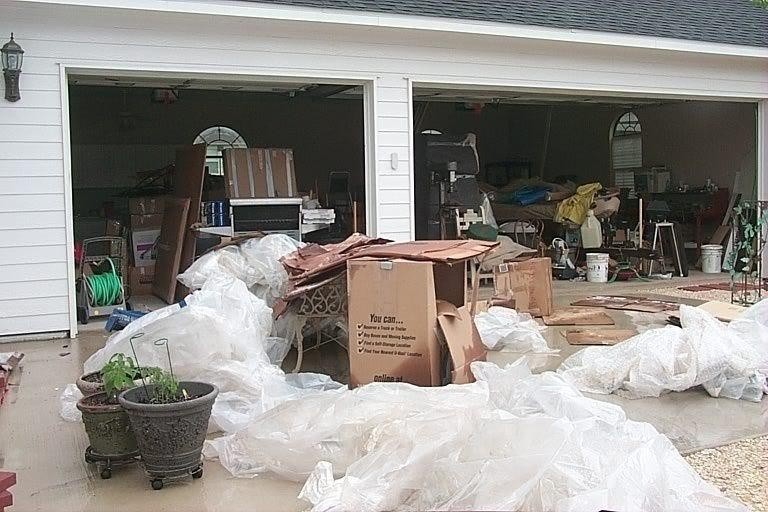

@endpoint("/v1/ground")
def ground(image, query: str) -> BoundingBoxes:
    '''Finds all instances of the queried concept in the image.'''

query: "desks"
[641,187,729,270]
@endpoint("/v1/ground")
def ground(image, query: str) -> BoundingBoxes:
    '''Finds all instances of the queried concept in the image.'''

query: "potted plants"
[75,333,221,490]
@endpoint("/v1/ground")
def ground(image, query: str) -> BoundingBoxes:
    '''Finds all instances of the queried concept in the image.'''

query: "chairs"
[455,206,547,295]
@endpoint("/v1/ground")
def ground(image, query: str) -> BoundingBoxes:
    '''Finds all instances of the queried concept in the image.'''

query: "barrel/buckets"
[580,210,603,248]
[698,245,723,273]
[587,253,609,283]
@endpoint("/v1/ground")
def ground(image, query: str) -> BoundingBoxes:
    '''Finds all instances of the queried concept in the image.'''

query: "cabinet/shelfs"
[71,145,183,190]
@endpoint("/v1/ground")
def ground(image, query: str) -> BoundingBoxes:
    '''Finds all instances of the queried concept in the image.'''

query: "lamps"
[150,88,180,105]
[0,30,24,101]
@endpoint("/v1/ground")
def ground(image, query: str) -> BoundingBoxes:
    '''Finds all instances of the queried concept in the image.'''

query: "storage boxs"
[346,257,552,388]
[107,194,172,295]
